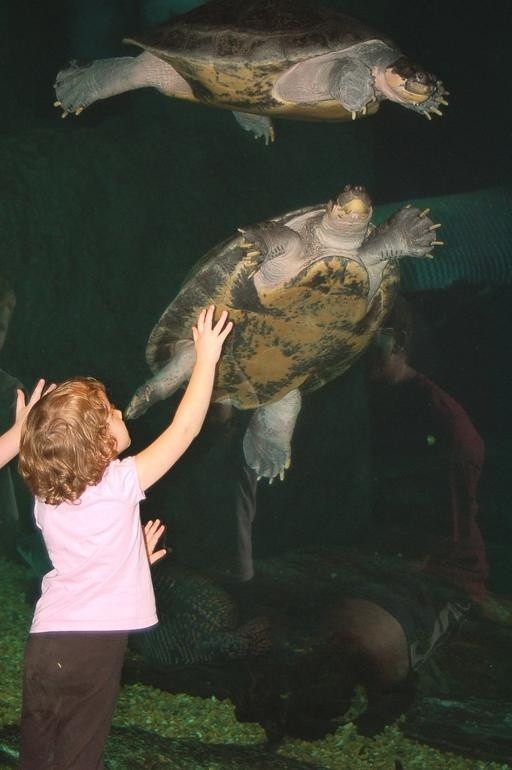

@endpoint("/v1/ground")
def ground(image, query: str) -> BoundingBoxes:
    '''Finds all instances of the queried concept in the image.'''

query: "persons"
[208,357,270,599]
[210,300,487,723]
[0,376,57,472]
[14,301,236,770]
